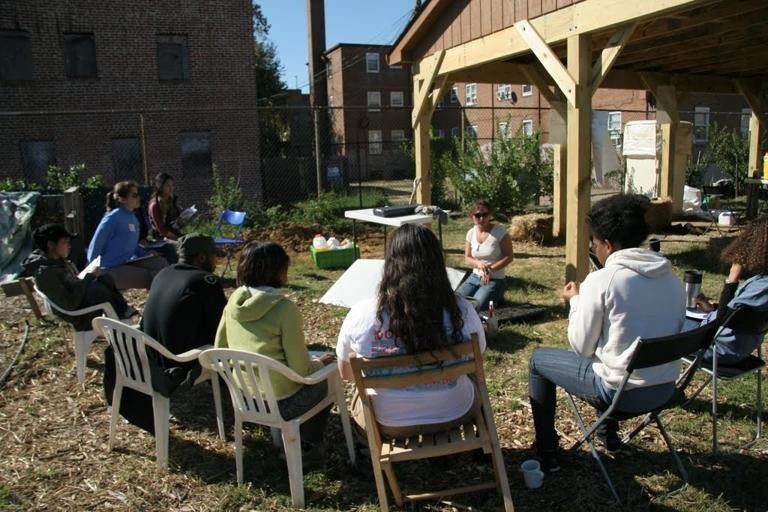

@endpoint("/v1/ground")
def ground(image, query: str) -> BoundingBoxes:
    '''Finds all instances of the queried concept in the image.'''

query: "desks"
[344,205,452,263]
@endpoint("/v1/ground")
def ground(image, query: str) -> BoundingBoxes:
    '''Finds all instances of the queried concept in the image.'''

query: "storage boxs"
[309,240,360,269]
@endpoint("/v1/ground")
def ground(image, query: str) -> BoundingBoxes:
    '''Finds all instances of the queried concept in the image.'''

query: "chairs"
[557,318,722,511]
[91,316,227,469]
[33,283,141,384]
[680,302,768,461]
[349,331,516,512]
[702,183,748,239]
[212,209,247,280]
[197,348,357,510]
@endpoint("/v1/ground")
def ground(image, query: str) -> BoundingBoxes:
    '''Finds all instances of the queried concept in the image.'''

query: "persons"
[213,242,336,458]
[148,173,186,264]
[679,217,768,367]
[86,181,169,291]
[454,200,512,313]
[20,224,138,331]
[336,223,487,481]
[530,195,686,470]
[142,232,227,387]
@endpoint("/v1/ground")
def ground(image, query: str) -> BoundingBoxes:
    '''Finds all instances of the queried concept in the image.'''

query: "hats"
[176,232,227,258]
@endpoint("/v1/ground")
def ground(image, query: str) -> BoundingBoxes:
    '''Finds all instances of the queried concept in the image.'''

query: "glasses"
[472,212,489,218]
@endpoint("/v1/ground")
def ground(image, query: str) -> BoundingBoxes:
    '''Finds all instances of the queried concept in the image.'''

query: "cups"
[683,270,702,309]
[521,459,545,490]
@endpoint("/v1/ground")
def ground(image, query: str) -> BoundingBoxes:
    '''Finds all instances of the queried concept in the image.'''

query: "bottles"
[484,300,497,337]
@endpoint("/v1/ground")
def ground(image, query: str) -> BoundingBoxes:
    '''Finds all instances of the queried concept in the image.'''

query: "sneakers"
[537,450,563,474]
[604,437,621,454]
